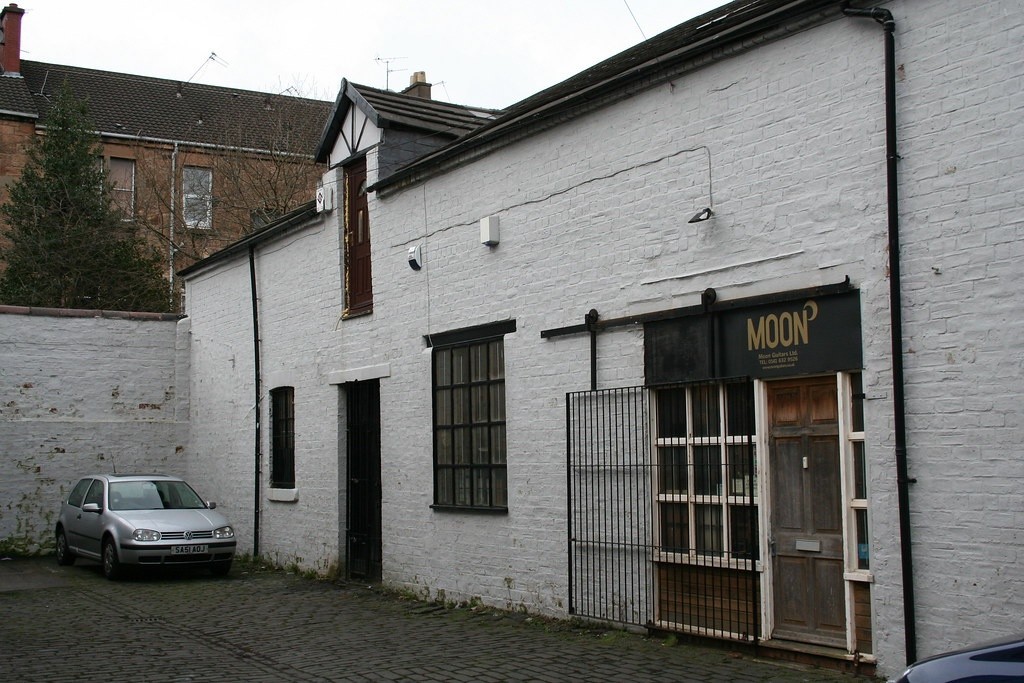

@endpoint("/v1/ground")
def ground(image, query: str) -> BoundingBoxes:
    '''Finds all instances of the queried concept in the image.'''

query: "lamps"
[687,207,713,222]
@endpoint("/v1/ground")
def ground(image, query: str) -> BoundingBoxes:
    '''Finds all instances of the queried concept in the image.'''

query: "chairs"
[100,490,123,509]
[144,489,166,509]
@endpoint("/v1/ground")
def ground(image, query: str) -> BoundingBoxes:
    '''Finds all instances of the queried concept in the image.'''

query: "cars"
[55,471,238,582]
